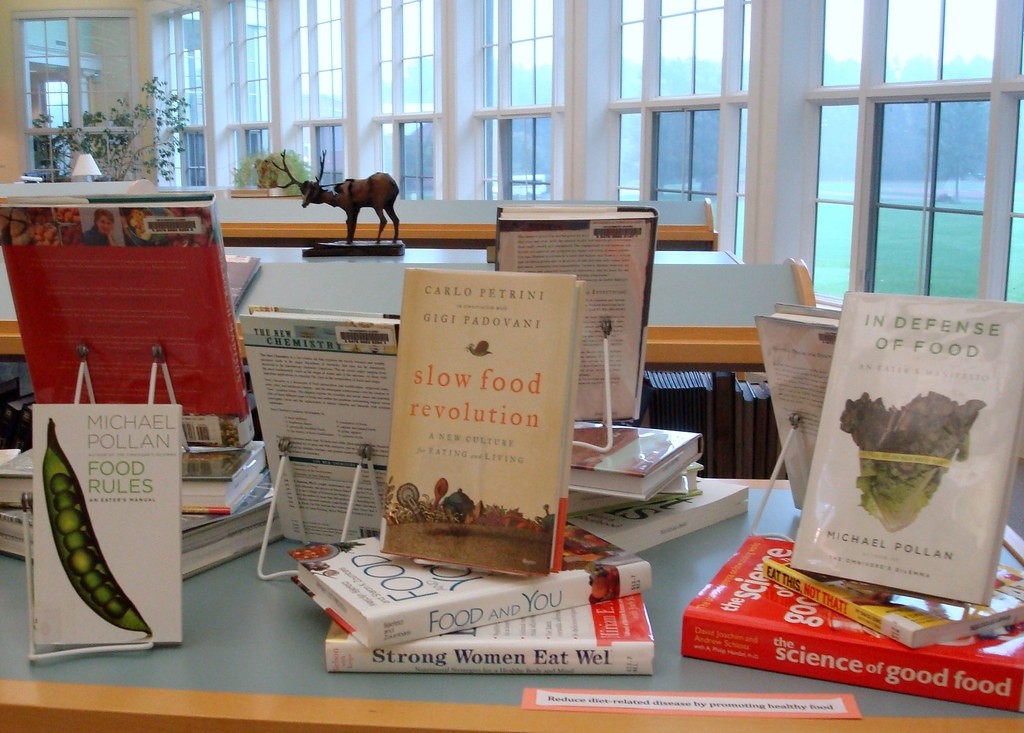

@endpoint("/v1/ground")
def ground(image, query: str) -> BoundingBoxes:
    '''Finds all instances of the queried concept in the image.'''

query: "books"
[322,591,661,675]
[288,514,653,651]
[28,397,182,646]
[495,203,658,420]
[378,267,590,577]
[681,534,1022,714]
[570,476,750,553]
[642,368,787,479]
[752,301,843,512]
[225,252,260,309]
[568,461,705,520]
[0,475,286,582]
[239,302,401,546]
[569,418,706,501]
[0,189,256,451]
[791,289,1024,607]
[762,552,1023,648]
[1,438,270,516]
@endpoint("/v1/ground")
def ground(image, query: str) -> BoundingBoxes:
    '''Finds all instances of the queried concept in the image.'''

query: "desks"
[0,474,1021,732]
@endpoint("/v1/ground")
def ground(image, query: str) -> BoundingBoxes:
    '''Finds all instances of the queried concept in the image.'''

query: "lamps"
[71,153,103,182]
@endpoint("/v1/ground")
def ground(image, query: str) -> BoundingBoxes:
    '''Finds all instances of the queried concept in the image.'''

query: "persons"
[82,207,118,245]
